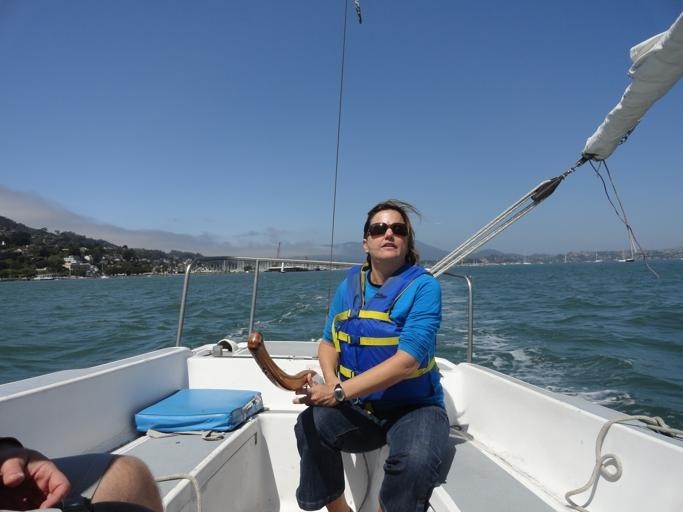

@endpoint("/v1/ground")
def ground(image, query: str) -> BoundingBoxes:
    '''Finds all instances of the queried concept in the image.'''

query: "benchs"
[427,426,571,512]
[105,408,281,511]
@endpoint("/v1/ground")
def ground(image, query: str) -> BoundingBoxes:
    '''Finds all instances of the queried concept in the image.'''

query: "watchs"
[333,383,345,403]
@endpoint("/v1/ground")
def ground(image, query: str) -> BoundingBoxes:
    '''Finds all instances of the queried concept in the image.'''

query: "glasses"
[365,222,411,238]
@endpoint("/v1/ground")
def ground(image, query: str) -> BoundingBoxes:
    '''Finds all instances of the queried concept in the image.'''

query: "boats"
[34,274,54,280]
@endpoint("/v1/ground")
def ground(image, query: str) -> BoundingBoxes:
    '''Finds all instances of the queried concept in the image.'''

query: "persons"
[291,198,450,512]
[0,435,164,512]
